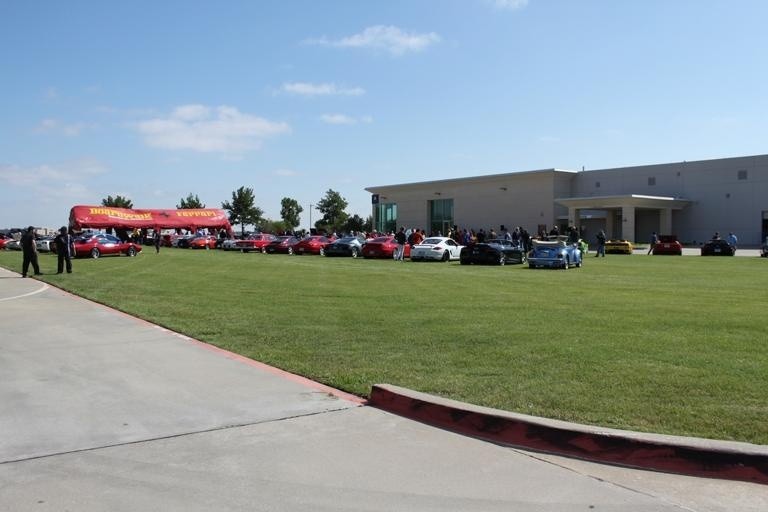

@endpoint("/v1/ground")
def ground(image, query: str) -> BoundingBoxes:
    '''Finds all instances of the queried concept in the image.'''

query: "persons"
[593,229,606,258]
[727,233,736,256]
[712,231,720,238]
[392,226,406,262]
[647,231,657,254]
[52,226,77,274]
[153,230,162,255]
[19,225,43,278]
[219,229,226,242]
[131,227,146,246]
[286,223,581,254]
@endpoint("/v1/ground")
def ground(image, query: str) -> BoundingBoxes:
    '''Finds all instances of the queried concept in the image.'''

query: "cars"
[266,236,299,255]
[654,235,683,256]
[409,237,467,263]
[2,236,23,250]
[37,236,58,254]
[144,229,207,249]
[362,236,412,259]
[757,238,768,258]
[236,234,278,254]
[701,239,736,256]
[295,235,332,255]
[457,237,528,268]
[324,237,365,259]
[73,233,142,257]
[222,237,243,251]
[190,235,222,249]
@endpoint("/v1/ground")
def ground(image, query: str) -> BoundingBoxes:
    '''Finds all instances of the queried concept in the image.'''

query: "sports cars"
[522,234,585,272]
[604,240,632,252]
